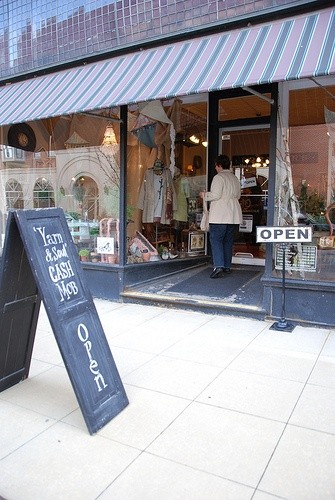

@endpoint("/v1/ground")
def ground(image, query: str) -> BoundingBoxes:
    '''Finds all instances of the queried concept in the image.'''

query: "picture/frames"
[188,231,206,257]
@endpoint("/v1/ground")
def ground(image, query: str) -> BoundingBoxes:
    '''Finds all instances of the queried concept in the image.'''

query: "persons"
[200,155,243,277]
[138,163,190,225]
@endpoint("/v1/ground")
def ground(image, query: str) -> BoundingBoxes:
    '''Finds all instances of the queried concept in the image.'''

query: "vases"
[142,252,151,262]
[107,255,116,263]
[90,252,98,263]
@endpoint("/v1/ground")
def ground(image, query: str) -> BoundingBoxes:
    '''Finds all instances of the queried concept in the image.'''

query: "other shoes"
[224,268,230,273]
[210,267,223,278]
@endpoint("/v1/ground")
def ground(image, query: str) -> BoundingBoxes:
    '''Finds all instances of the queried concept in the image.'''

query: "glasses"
[214,163,218,168]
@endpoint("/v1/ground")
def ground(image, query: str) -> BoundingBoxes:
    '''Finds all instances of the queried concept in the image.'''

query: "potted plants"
[79,249,89,262]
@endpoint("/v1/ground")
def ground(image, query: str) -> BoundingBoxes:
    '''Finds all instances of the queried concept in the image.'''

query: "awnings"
[0,11,335,126]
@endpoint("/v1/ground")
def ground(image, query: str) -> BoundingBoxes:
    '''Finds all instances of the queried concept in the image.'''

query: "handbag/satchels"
[199,192,210,232]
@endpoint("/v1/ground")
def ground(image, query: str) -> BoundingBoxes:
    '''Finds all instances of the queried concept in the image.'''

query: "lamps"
[189,131,207,147]
[245,155,269,168]
[100,110,119,155]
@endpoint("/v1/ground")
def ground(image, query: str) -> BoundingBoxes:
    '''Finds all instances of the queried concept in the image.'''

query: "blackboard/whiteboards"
[0,205,130,436]
